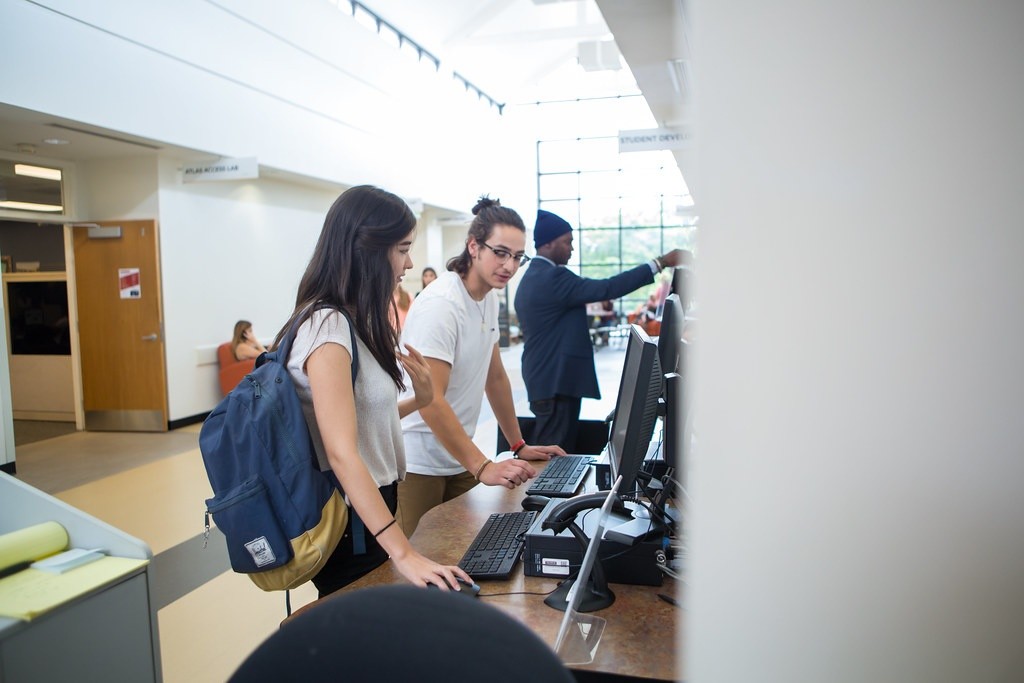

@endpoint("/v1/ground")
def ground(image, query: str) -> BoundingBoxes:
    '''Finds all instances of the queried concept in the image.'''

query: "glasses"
[478,239,531,267]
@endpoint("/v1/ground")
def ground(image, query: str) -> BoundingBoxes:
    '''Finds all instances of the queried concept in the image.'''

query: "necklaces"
[463,275,487,332]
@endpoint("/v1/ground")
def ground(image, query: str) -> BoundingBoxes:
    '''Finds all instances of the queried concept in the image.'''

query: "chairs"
[224,584,575,683]
[587,312,640,351]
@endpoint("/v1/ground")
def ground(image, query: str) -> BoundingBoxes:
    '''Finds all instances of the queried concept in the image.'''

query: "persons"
[196,186,475,601]
[588,299,616,346]
[223,585,575,683]
[391,198,566,540]
[414,267,437,297]
[636,275,671,325]
[513,210,693,454]
[230,320,269,361]
[388,283,413,348]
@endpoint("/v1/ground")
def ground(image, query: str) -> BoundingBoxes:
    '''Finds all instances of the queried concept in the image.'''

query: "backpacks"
[199,299,359,592]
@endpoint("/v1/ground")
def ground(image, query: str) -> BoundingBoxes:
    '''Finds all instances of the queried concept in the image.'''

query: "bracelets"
[374,519,397,537]
[475,459,492,480]
[509,439,526,456]
[653,256,665,273]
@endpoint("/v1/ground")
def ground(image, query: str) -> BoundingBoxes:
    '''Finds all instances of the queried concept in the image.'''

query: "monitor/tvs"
[606,265,683,545]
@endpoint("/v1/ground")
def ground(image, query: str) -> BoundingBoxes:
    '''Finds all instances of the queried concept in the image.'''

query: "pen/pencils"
[653,593,684,607]
[0,559,36,581]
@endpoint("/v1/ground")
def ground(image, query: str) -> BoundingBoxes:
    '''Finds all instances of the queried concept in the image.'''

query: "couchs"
[218,342,256,397]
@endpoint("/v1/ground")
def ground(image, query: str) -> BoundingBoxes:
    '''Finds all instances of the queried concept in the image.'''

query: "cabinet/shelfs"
[0,469,163,683]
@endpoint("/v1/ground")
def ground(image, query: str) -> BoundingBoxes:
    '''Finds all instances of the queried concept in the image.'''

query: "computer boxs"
[524,497,666,587]
[595,441,678,500]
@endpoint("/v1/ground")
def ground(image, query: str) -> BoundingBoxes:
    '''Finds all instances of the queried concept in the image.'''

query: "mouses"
[424,574,480,597]
[521,495,550,511]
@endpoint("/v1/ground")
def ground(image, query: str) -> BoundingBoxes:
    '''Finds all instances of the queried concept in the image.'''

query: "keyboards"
[454,508,538,580]
[525,456,594,496]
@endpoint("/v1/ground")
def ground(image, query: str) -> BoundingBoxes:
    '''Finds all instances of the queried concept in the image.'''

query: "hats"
[534,210,573,248]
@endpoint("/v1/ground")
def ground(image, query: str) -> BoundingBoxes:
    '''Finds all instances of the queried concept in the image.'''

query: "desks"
[279,455,681,681]
